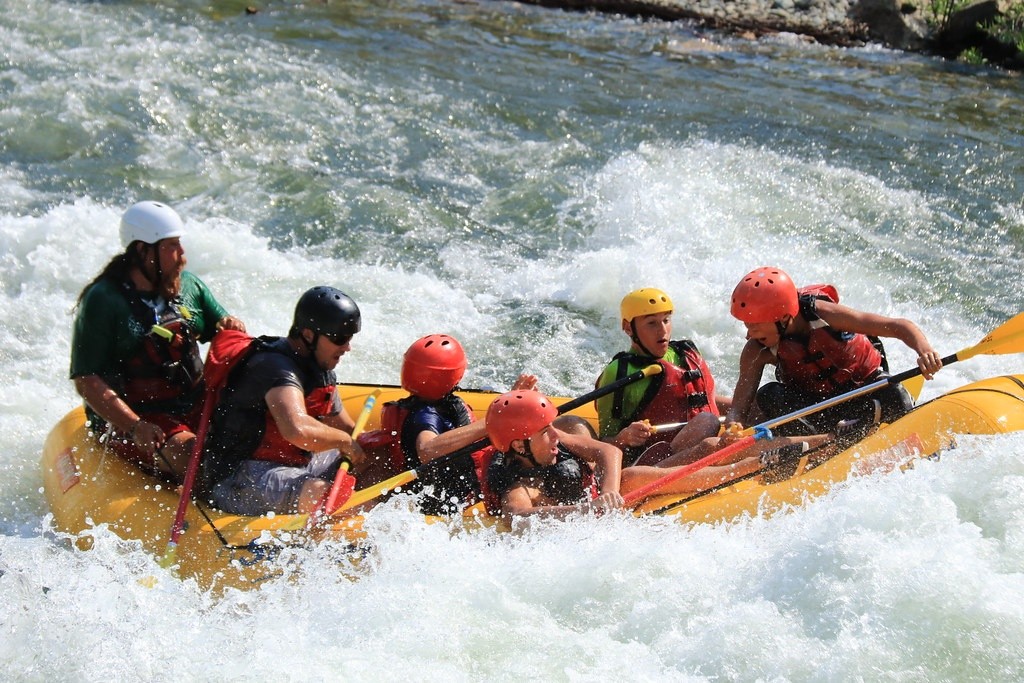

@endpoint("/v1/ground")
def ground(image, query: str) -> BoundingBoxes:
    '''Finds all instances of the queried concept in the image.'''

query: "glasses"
[321,332,353,346]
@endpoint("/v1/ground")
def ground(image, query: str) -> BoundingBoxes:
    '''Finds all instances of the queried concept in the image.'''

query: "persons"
[592,286,765,477]
[719,267,944,480]
[67,201,248,484]
[193,285,370,518]
[484,389,890,546]
[391,335,537,520]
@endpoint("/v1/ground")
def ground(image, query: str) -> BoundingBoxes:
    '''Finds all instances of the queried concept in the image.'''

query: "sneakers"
[758,442,810,486]
[835,399,882,440]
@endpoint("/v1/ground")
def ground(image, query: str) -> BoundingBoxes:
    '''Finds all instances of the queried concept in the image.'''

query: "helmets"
[294,286,361,336]
[621,288,673,330]
[485,389,558,452]
[119,200,183,247]
[401,334,466,399]
[731,268,800,324]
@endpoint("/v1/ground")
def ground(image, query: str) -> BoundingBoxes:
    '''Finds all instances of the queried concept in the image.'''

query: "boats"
[46,313,1023,595]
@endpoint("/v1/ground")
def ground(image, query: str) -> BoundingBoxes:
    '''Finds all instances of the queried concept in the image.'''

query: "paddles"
[639,416,728,435]
[144,384,216,586]
[333,365,663,513]
[305,389,382,530]
[732,311,1023,438]
[592,426,771,518]
